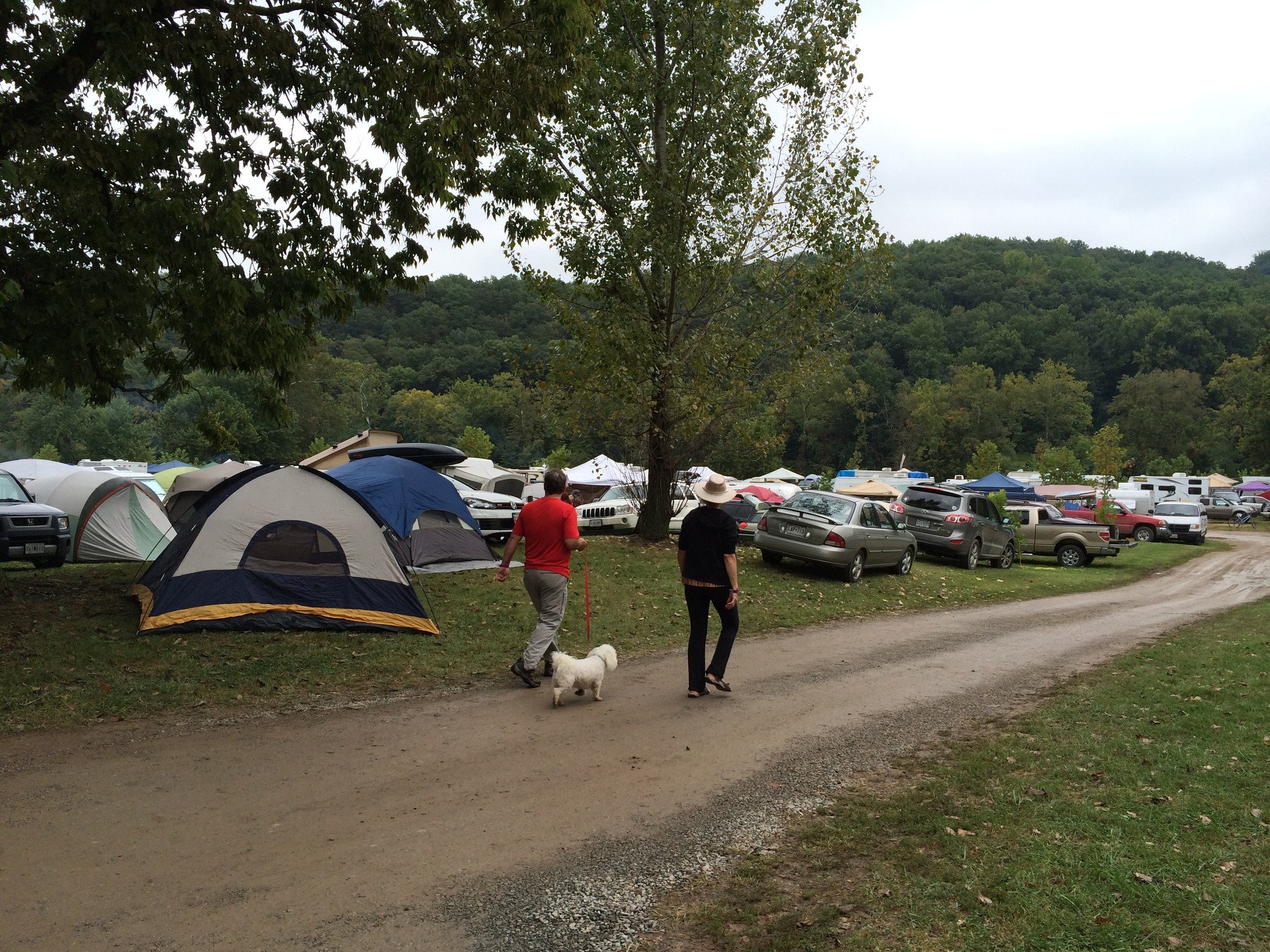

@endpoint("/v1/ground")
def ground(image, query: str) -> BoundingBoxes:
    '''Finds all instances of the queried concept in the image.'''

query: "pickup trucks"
[1196,494,1258,523]
[1002,498,1138,567]
[1058,499,1172,543]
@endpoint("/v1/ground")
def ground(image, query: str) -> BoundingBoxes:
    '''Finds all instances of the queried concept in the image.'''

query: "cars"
[1240,496,1270,514]
[1259,506,1270,519]
[796,480,817,490]
[752,489,918,583]
[435,472,803,547]
[1212,492,1244,505]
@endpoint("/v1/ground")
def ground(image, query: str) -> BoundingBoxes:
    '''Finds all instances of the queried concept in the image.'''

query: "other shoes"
[688,688,710,699]
[706,674,732,692]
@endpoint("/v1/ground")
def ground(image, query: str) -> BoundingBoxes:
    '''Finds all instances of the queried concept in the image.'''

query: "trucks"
[75,465,167,502]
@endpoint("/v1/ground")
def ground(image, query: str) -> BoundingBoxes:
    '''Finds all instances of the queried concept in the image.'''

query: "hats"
[694,472,737,503]
[570,489,579,494]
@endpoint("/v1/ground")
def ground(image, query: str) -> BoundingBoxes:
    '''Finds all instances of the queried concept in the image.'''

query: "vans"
[1147,500,1208,546]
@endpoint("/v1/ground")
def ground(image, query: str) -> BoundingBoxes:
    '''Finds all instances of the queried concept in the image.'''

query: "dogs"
[549,644,618,708]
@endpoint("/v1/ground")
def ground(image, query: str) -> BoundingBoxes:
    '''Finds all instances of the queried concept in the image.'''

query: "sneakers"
[510,656,541,687]
[544,663,554,676]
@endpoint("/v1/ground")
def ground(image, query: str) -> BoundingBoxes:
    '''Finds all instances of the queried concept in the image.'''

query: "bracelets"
[729,589,739,593]
[498,564,509,568]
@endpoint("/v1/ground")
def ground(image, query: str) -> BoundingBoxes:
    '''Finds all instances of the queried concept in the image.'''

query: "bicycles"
[1228,510,1257,529]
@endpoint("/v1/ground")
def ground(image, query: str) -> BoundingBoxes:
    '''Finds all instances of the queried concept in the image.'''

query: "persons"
[571,489,584,508]
[560,492,572,505]
[677,474,739,698]
[495,468,587,687]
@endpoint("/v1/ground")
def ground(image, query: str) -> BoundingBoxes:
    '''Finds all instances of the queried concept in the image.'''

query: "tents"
[323,455,499,567]
[682,466,730,483]
[760,468,806,490]
[1208,472,1270,504]
[732,485,787,503]
[0,460,232,561]
[562,454,645,486]
[122,464,444,637]
[836,471,1046,503]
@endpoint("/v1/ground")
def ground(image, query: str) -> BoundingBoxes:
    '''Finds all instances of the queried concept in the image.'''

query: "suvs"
[0,469,72,568]
[888,482,1016,571]
